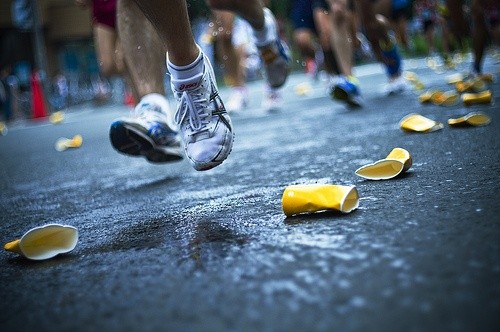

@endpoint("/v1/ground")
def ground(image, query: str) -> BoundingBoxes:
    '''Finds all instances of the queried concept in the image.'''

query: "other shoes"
[264,88,284,111]
[227,89,247,114]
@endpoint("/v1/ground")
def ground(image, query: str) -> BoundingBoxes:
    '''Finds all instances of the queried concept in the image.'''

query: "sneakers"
[253,8,291,88]
[333,77,364,110]
[380,43,402,79]
[170,54,236,172]
[109,105,184,165]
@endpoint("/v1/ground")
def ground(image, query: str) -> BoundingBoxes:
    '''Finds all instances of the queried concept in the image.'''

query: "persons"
[75,0,500,170]
